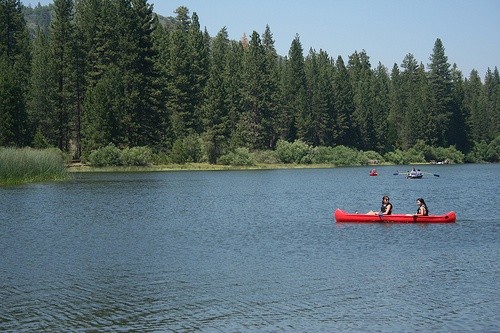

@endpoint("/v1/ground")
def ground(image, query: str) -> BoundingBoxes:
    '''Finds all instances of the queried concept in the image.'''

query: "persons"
[410,167,422,175]
[407,198,429,216]
[367,196,392,215]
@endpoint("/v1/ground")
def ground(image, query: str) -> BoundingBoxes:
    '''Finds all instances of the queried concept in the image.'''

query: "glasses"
[386,199,389,201]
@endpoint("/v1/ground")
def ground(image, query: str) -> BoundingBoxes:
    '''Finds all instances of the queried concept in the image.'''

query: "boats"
[407,173,424,178]
[370,173,378,176]
[392,173,399,175]
[333,209,457,223]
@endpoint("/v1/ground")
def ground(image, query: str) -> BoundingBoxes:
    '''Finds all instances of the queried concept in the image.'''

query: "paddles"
[393,170,409,175]
[378,213,384,216]
[422,172,440,177]
[413,214,418,217]
